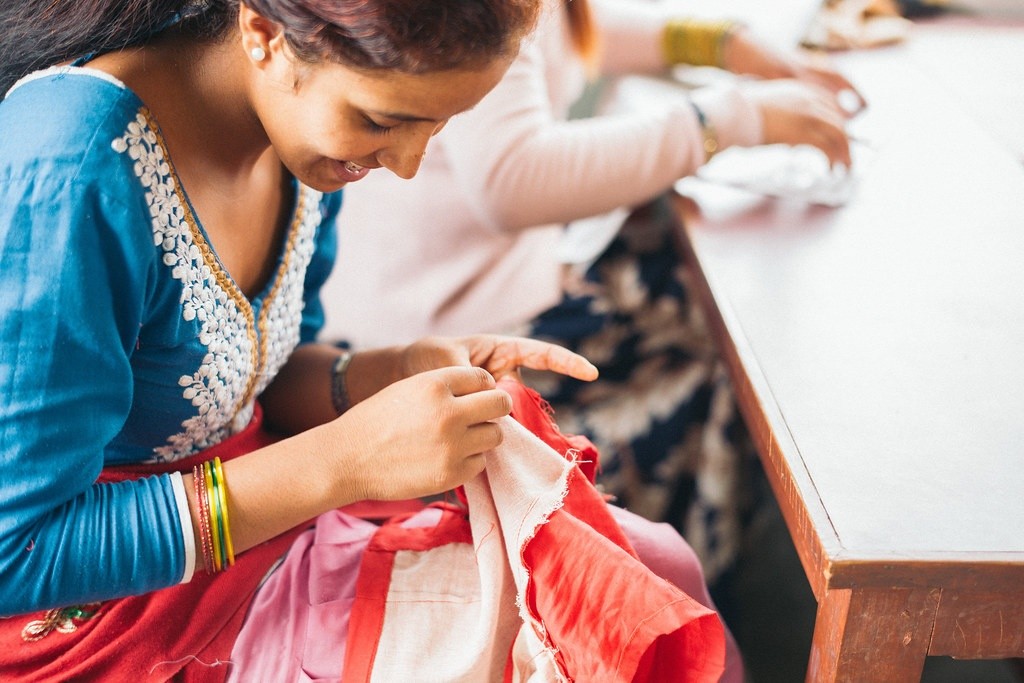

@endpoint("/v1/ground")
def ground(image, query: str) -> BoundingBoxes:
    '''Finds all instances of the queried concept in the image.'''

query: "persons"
[316,1,870,351]
[0,0,742,683]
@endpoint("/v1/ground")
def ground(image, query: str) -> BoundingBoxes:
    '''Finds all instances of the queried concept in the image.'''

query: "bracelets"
[688,99,716,168]
[331,352,359,416]
[193,456,236,576]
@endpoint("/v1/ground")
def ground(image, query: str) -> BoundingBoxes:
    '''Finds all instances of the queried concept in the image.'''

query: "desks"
[670,18,1024,683]
